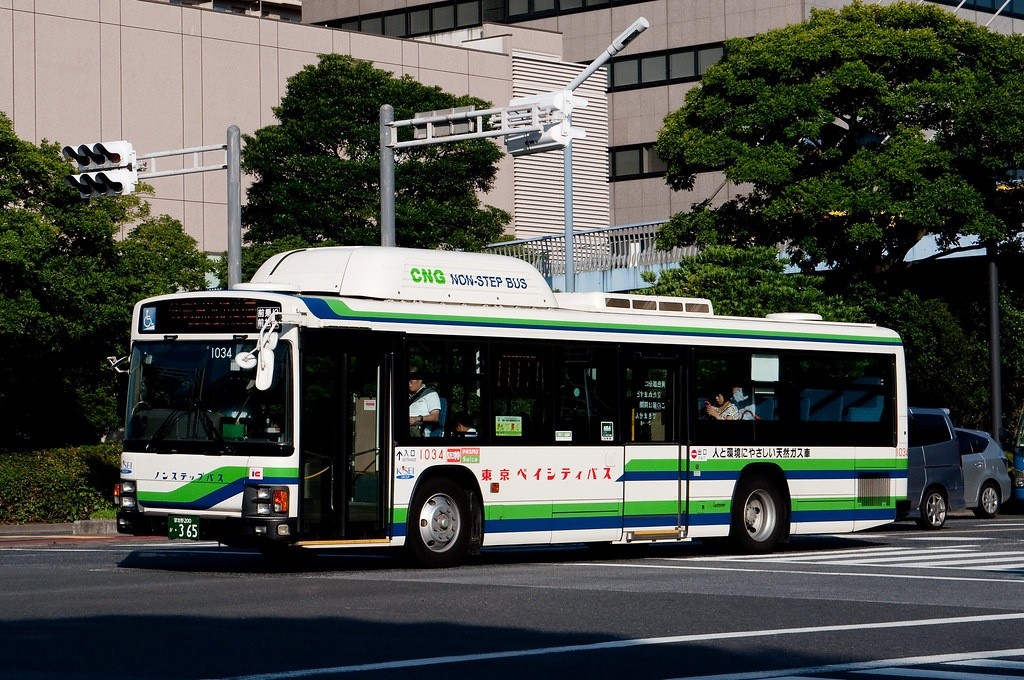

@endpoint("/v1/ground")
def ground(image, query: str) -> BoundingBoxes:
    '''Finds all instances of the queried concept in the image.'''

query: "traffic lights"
[59,141,136,170]
[64,171,138,199]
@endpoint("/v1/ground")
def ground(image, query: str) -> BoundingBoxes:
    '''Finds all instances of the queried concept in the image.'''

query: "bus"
[106,242,908,570]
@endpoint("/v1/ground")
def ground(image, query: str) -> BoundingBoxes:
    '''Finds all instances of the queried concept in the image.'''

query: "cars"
[953,427,1012,519]
[909,405,966,530]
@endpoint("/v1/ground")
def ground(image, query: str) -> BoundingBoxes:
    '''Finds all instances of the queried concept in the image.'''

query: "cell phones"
[703,402,709,406]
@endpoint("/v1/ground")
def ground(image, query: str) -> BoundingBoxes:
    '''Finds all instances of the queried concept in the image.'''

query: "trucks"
[1009,409,1024,505]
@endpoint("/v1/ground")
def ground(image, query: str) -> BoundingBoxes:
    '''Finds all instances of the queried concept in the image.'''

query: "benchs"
[697,376,886,423]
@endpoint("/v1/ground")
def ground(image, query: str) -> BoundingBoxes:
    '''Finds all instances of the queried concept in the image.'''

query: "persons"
[705,393,738,421]
[408,365,441,438]
[451,412,479,437]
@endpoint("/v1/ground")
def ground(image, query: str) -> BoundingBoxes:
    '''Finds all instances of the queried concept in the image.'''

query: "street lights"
[564,16,649,293]
[984,178,1024,441]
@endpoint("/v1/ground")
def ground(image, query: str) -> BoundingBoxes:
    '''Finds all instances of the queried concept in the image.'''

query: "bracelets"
[418,415,423,422]
